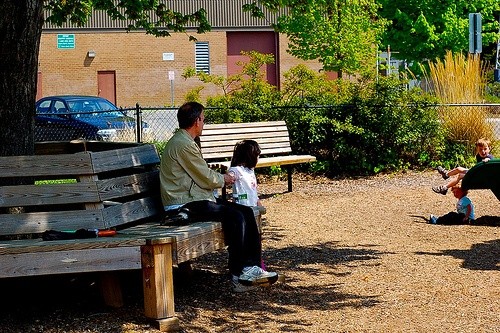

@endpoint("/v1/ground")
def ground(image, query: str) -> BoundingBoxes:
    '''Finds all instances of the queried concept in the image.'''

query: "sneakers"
[432,185,448,195]
[437,165,450,180]
[238,265,279,288]
[231,279,257,292]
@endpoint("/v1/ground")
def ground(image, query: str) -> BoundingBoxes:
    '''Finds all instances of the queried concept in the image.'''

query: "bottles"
[238,185,248,206]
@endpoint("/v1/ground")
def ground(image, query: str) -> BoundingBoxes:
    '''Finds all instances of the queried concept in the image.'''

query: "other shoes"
[430,214,438,224]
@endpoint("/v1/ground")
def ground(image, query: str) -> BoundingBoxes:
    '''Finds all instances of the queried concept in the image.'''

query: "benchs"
[39,107,73,114]
[0,145,267,333]
[193,120,317,198]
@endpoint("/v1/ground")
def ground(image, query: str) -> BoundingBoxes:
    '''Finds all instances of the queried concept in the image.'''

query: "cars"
[32,95,153,142]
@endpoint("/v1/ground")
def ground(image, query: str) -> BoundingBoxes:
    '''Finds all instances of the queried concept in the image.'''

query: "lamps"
[88,51,96,57]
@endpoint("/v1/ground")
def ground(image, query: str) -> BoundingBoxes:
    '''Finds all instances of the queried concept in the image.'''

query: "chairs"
[82,106,94,116]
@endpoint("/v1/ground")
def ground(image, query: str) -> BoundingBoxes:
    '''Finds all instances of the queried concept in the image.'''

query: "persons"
[432,137,493,195]
[430,181,474,225]
[226,140,263,206]
[159,102,278,291]
